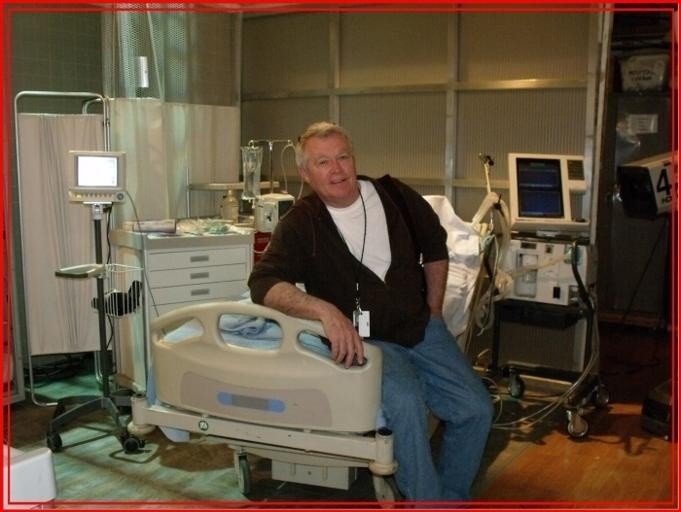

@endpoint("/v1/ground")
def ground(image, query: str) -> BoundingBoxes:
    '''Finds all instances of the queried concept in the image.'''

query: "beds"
[146,220,496,501]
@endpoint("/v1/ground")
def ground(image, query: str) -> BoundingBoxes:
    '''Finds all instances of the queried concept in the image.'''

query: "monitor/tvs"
[508,152,585,221]
[68,150,127,193]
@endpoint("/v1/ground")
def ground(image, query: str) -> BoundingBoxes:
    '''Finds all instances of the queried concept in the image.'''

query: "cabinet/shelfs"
[111,224,259,398]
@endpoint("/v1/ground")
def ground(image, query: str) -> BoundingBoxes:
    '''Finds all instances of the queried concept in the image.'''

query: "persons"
[247,122,496,504]
